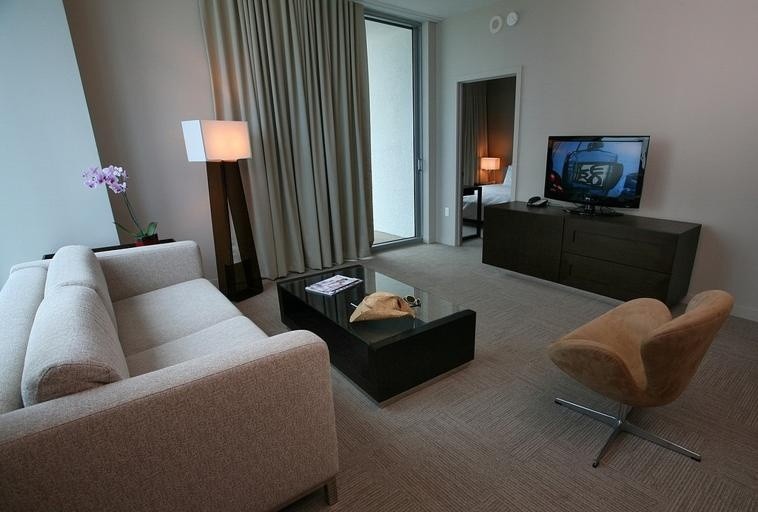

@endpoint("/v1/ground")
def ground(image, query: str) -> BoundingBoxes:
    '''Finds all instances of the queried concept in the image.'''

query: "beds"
[462,165,512,222]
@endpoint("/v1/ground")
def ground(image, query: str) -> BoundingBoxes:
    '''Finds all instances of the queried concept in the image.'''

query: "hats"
[350,292,415,322]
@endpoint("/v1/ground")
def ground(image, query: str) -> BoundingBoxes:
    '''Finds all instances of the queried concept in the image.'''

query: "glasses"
[402,295,421,307]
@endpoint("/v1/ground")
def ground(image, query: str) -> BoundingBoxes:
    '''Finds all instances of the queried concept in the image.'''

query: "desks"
[461,186,482,242]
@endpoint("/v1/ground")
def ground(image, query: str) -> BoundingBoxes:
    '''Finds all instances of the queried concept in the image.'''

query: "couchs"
[0,240,340,512]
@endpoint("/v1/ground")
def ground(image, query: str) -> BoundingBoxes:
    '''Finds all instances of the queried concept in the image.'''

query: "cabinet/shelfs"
[482,200,702,309]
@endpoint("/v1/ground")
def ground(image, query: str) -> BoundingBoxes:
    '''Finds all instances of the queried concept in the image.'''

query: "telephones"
[527,196,548,207]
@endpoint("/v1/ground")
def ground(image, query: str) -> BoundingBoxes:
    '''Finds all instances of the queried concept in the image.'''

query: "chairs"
[548,290,735,468]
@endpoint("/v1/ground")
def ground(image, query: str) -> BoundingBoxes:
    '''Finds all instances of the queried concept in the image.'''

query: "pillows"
[21,285,130,407]
[44,246,118,332]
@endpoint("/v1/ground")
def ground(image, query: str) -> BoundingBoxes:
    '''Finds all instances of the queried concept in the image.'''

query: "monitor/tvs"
[544,136,650,216]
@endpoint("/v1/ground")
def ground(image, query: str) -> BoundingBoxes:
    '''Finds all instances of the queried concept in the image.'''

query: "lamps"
[480,157,500,184]
[181,119,264,302]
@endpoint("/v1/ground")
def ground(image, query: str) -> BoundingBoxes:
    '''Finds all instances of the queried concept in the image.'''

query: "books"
[305,274,364,297]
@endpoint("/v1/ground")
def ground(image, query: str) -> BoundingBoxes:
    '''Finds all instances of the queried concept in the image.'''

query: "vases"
[135,235,159,246]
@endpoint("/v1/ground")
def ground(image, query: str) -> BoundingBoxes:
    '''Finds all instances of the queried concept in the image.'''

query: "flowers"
[80,165,159,240]
